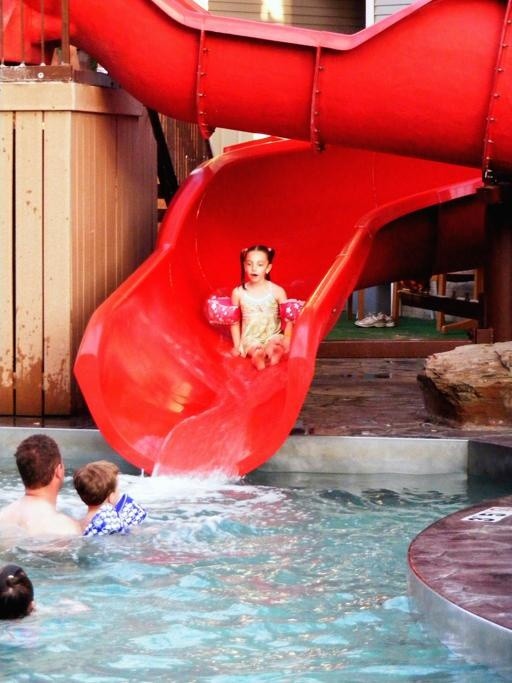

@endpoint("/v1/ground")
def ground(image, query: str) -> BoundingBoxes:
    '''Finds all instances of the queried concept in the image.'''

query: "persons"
[1,562,36,620]
[226,243,292,369]
[74,458,152,534]
[0,434,80,554]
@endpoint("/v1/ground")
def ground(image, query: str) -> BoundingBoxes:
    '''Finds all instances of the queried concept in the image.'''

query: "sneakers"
[354,312,385,328]
[378,311,395,326]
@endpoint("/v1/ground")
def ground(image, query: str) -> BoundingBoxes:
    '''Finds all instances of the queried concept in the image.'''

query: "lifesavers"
[203,297,305,324]
[82,494,147,536]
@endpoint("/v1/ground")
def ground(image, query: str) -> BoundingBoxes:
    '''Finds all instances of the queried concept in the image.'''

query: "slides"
[0,1,512,480]
